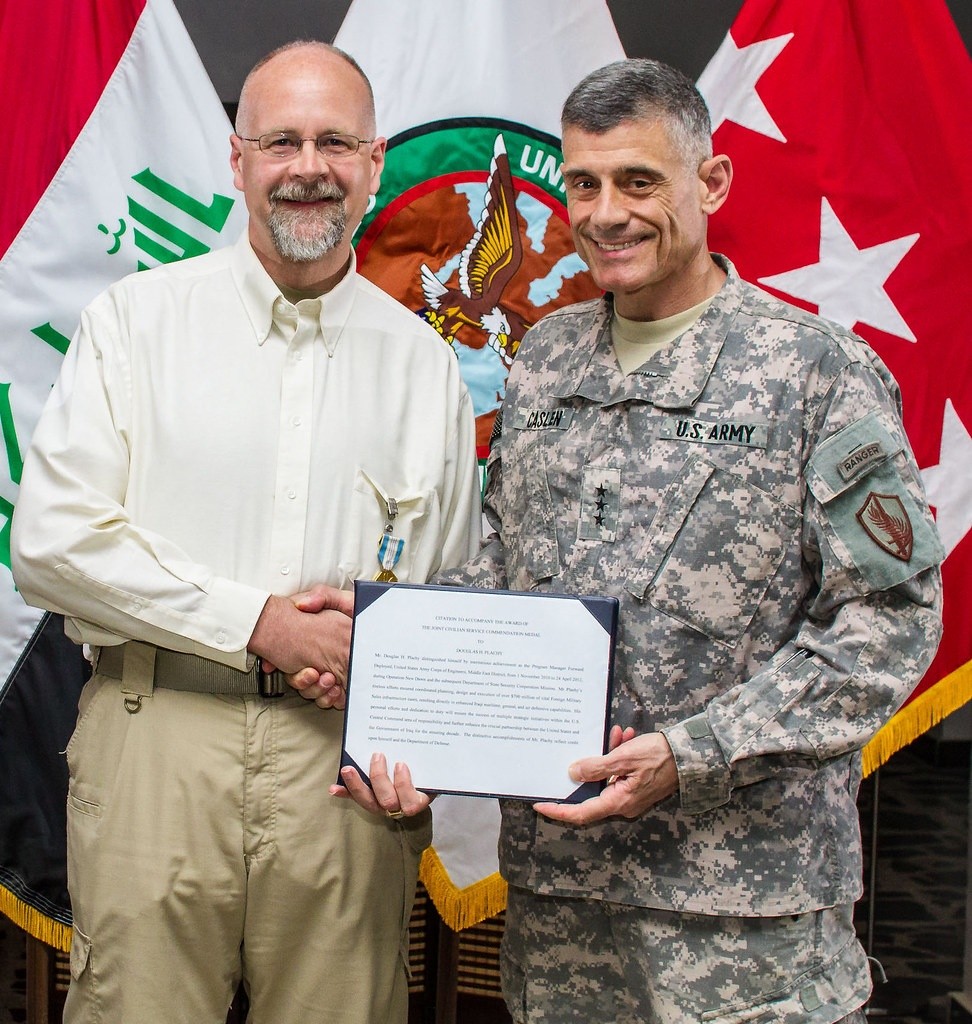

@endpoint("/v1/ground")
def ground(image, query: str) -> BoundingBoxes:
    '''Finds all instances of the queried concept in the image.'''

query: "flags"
[1,0,972,955]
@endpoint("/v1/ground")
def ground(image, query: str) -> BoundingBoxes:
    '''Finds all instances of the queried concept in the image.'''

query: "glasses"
[244,131,373,156]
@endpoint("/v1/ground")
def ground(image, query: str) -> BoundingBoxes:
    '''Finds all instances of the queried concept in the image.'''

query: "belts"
[98,641,288,696]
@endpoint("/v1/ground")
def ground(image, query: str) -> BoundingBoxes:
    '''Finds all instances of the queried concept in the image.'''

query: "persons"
[262,57,944,1024]
[10,39,482,1024]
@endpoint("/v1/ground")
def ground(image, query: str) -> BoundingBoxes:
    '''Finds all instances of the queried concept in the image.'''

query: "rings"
[385,808,406,820]
